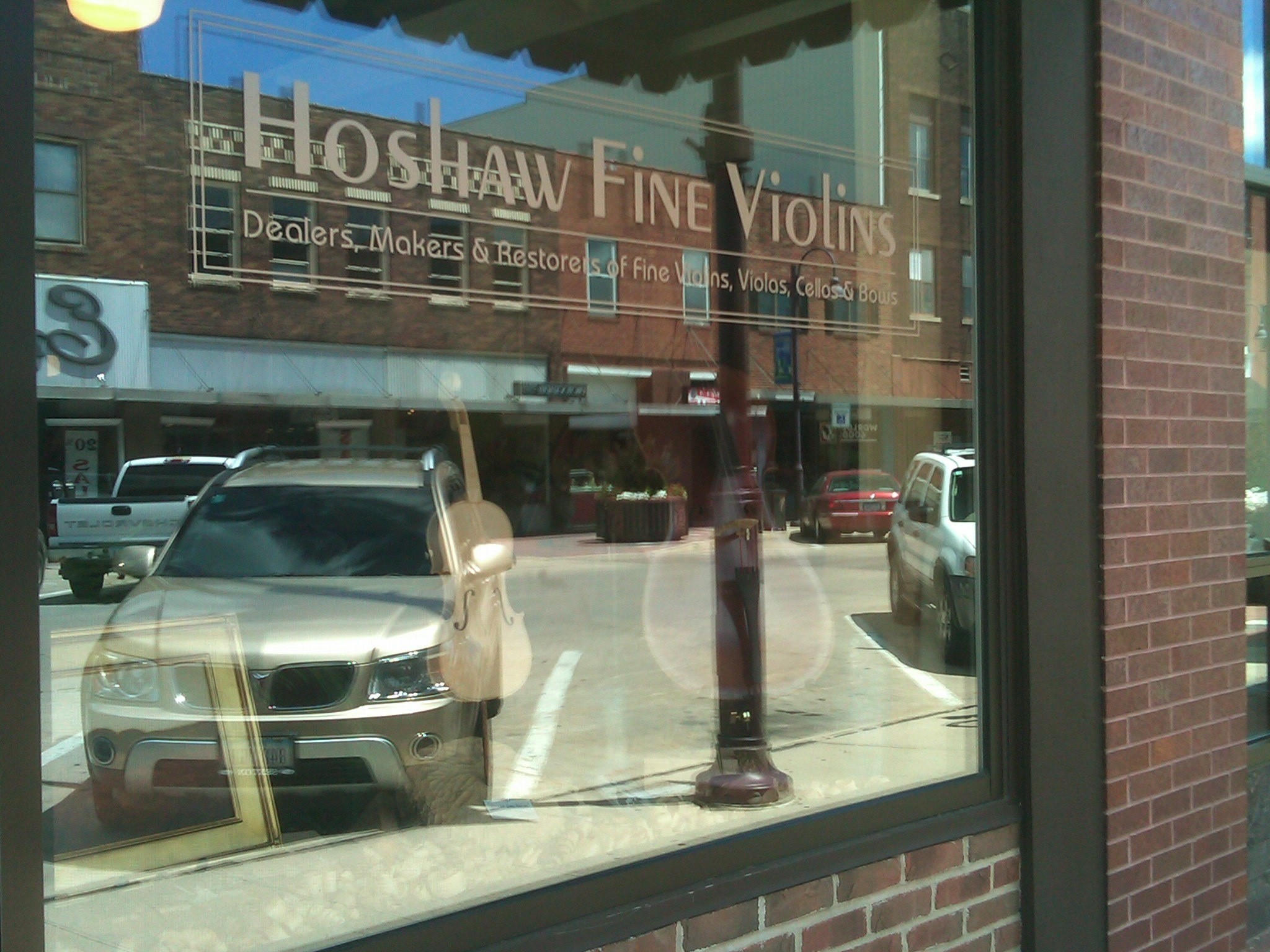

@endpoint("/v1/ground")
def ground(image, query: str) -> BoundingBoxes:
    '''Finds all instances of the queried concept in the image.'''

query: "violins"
[427,369,537,701]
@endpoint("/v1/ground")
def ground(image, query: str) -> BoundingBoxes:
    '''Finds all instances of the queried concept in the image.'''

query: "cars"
[797,468,904,544]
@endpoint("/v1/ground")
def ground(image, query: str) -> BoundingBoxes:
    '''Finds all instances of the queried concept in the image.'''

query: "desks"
[595,496,689,543]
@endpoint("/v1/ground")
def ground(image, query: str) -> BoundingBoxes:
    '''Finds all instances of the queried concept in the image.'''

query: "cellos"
[635,287,839,691]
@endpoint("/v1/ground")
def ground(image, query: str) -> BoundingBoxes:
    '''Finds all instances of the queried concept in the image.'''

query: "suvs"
[884,446,979,674]
[77,439,505,832]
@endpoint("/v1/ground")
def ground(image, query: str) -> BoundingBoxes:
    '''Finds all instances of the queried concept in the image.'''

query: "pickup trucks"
[46,454,235,601]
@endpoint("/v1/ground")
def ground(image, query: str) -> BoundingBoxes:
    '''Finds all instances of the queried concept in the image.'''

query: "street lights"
[789,246,846,527]
